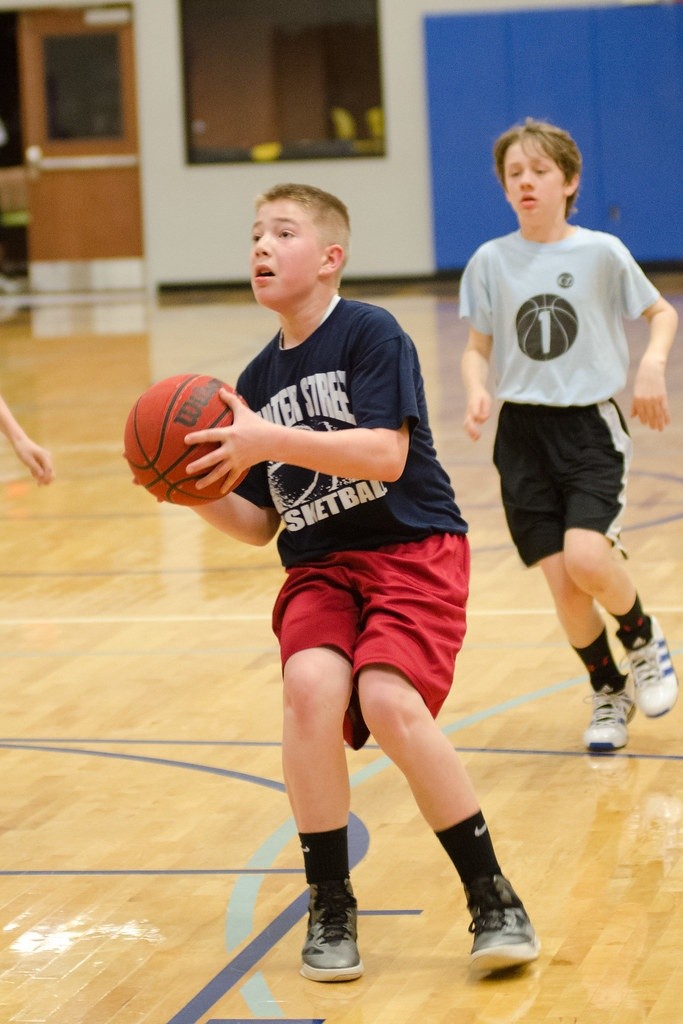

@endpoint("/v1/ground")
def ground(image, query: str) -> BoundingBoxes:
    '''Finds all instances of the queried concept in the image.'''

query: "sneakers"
[460,873,539,980]
[622,614,678,717]
[582,673,635,752]
[298,878,363,981]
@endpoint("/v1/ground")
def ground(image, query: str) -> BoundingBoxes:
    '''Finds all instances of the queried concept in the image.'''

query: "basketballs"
[125,373,252,506]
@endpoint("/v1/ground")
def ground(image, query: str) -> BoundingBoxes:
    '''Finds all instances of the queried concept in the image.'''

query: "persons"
[1,396,56,486]
[121,181,539,981]
[459,117,679,751]
